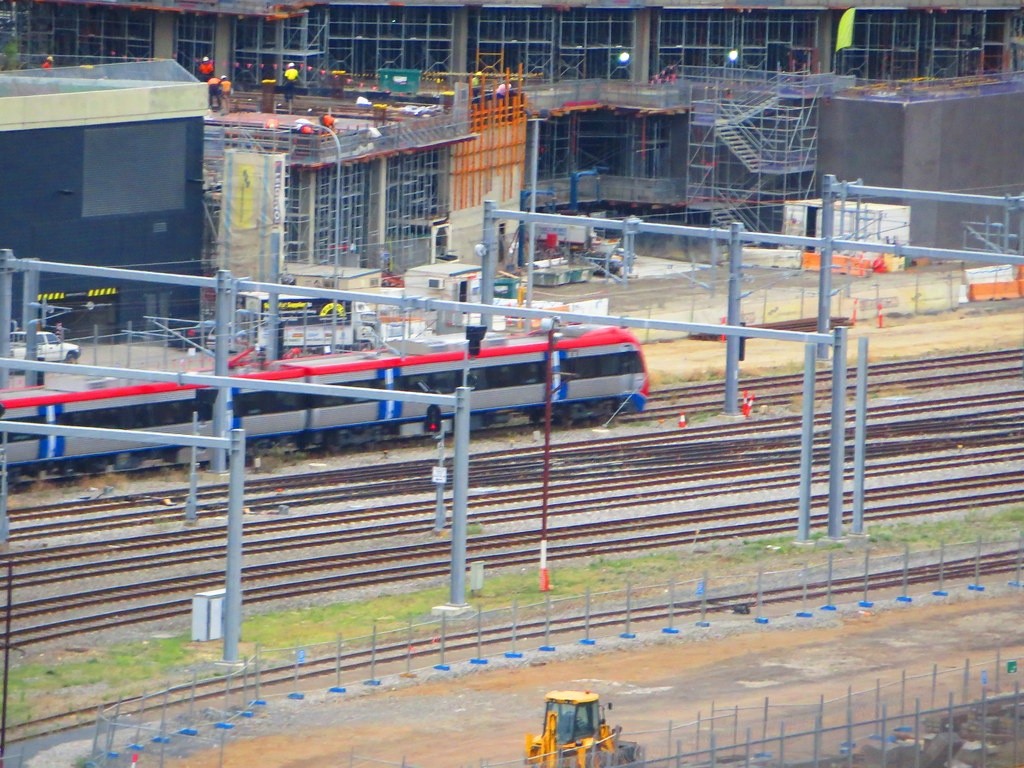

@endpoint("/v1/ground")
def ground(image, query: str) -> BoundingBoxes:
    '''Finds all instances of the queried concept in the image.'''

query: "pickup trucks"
[10,331,80,375]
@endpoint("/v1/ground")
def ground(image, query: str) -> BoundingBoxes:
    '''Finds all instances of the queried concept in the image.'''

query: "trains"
[0,319,653,484]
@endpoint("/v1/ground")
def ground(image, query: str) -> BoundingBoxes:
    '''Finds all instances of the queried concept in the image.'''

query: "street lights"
[295,118,342,355]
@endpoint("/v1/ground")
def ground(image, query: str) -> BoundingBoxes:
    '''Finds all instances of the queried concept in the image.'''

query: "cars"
[170,319,246,349]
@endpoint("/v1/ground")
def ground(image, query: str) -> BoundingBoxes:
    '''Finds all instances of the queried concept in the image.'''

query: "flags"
[835,7,856,51]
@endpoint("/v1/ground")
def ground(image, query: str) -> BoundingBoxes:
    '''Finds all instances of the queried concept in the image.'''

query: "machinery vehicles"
[521,689,643,768]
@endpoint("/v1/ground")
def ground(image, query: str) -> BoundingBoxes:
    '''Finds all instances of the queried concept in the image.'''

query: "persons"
[199,56,214,83]
[42,56,53,68]
[208,72,232,112]
[284,62,299,102]
[472,71,482,98]
[497,79,512,99]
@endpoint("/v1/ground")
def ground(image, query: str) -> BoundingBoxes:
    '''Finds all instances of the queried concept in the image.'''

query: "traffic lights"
[426,404,442,433]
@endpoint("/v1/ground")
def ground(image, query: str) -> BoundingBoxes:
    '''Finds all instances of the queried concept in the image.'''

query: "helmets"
[202,57,209,61]
[47,57,54,62]
[221,75,227,79]
[476,72,482,76]
[288,62,294,67]
